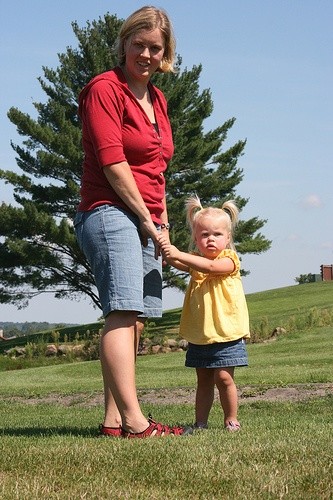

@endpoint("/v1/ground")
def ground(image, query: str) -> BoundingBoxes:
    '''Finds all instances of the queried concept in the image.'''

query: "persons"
[161,192,253,436]
[65,4,188,442]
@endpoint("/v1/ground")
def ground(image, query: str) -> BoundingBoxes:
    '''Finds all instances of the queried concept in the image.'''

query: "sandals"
[224,419,241,431]
[191,422,209,430]
[119,418,192,439]
[99,424,123,437]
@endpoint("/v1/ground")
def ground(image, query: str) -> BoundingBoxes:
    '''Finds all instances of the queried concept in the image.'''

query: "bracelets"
[157,222,172,230]
